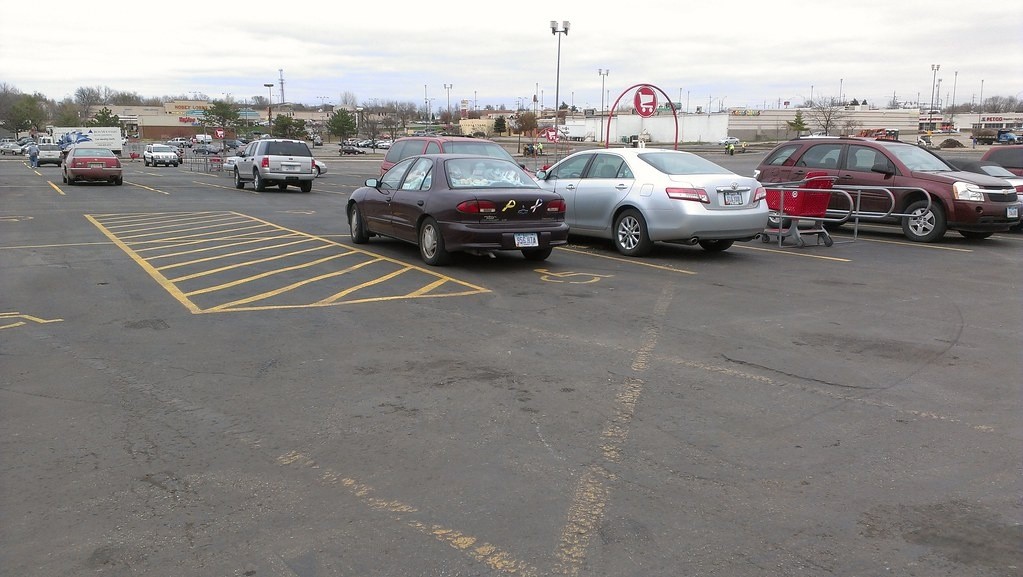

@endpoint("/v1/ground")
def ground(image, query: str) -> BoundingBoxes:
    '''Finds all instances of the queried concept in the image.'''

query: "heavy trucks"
[969,128,1023,146]
[552,123,586,142]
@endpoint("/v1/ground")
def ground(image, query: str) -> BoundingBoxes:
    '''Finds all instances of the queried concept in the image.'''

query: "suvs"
[379,135,534,179]
[233,139,316,192]
[143,143,179,168]
[753,134,1023,244]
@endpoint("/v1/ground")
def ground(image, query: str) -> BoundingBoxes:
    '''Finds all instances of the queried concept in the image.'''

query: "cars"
[345,154,571,266]
[809,131,830,136]
[305,125,453,156]
[60,145,124,186]
[718,137,739,145]
[0,126,130,167]
[166,134,252,171]
[315,160,328,178]
[532,147,769,258]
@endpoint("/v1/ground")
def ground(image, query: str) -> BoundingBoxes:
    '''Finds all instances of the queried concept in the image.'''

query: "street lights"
[928,64,941,131]
[316,96,329,127]
[598,68,609,142]
[549,19,571,164]
[263,83,274,138]
[443,83,453,136]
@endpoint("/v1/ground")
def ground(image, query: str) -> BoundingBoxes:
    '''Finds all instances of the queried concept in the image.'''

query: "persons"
[728,144,734,155]
[538,143,543,154]
[724,143,728,154]
[28,143,40,169]
[533,143,538,154]
[741,142,746,153]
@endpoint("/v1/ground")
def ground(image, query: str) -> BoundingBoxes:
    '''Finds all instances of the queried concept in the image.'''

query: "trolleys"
[758,171,840,247]
